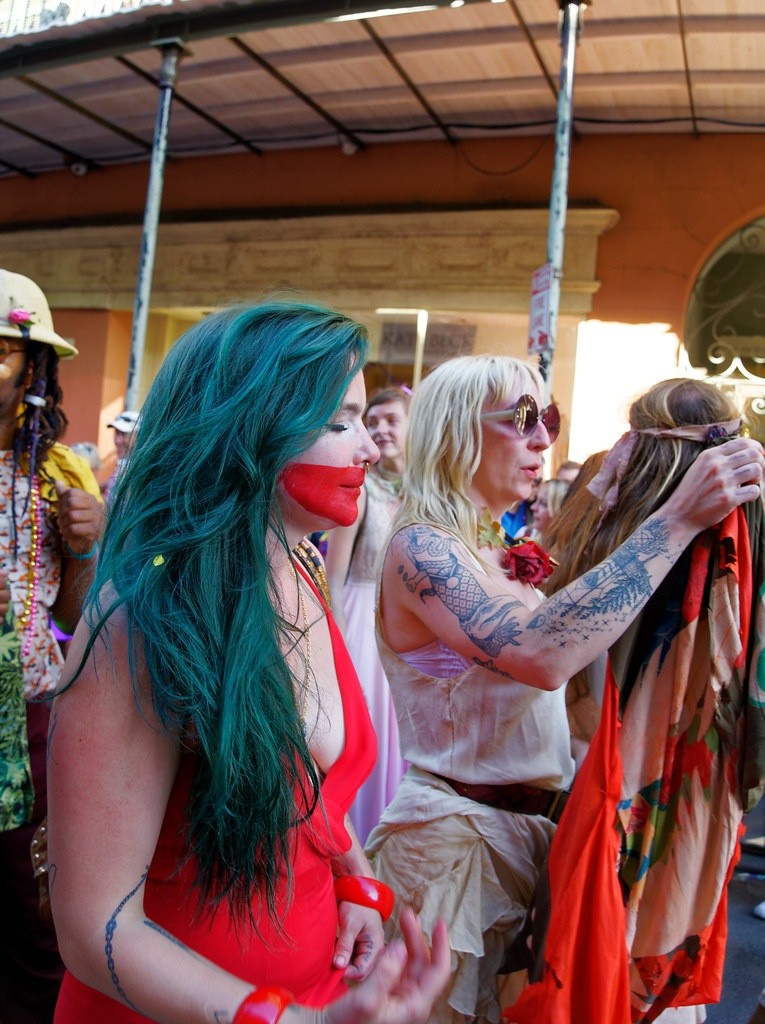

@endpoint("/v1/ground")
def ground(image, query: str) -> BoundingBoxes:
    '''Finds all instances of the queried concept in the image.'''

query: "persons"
[325,386,412,849]
[360,354,765,1024]
[0,268,106,1024]
[46,299,452,1024]
[499,378,765,1024]
[108,410,142,481]
[500,460,584,546]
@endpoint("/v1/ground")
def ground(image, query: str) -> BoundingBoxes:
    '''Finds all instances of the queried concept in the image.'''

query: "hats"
[0,269,79,359]
[107,411,143,433]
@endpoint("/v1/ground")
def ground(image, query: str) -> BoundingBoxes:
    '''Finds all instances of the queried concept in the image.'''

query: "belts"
[425,769,572,825]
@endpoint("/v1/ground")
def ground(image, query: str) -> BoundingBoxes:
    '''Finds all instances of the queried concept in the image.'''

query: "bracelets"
[231,984,293,1024]
[334,874,395,923]
[66,542,97,559]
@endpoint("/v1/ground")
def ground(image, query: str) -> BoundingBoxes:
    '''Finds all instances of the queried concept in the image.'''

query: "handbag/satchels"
[0,565,35,833]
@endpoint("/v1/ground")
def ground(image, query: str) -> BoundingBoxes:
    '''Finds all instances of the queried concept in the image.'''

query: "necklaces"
[18,475,41,657]
[286,558,311,722]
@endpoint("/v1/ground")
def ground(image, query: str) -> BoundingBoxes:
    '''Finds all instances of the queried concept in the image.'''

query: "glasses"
[0,338,27,364]
[479,395,561,444]
